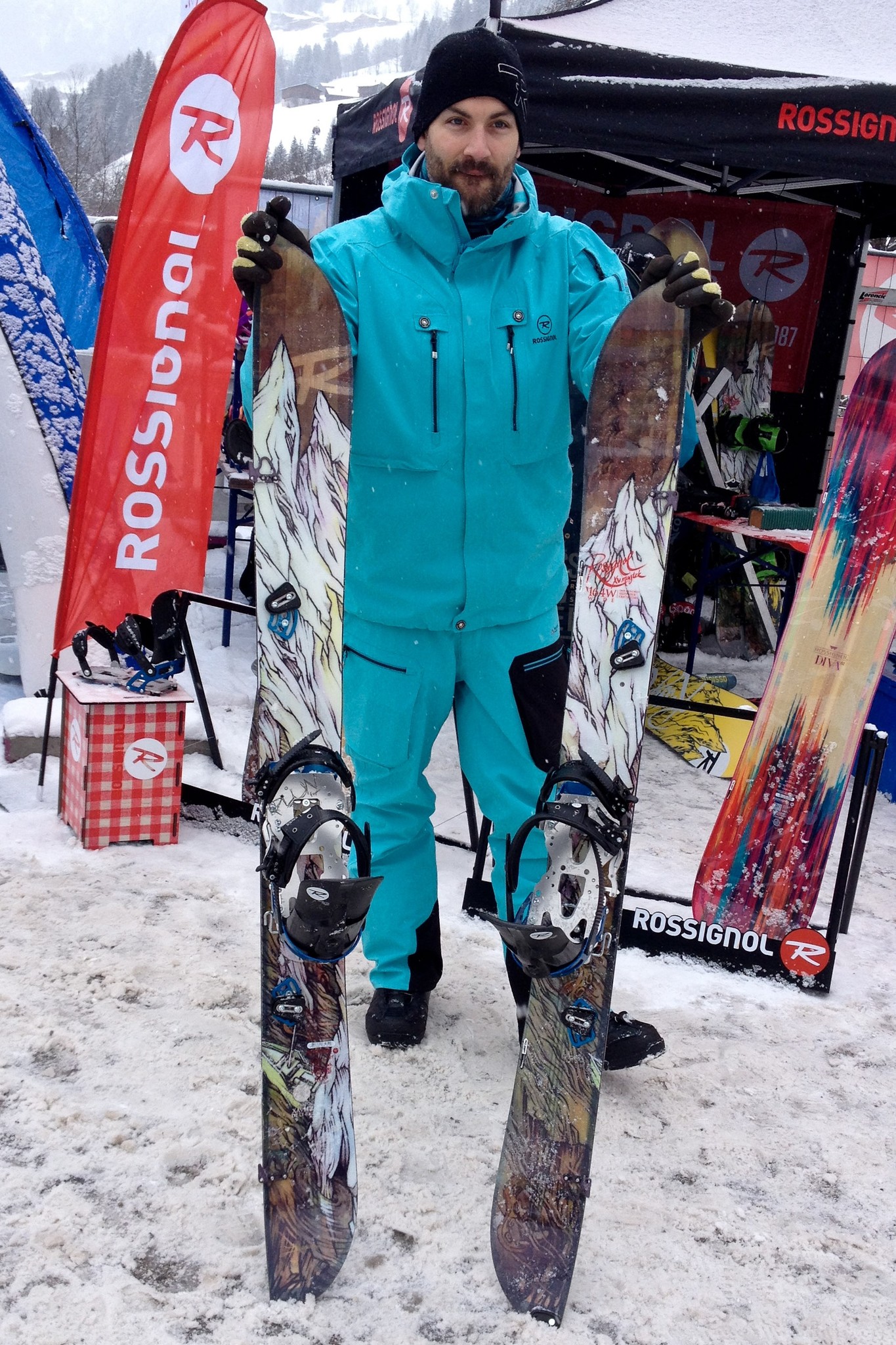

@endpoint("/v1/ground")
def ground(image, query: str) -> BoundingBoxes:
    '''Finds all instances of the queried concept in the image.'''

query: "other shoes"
[365,986,432,1053]
[519,1012,666,1072]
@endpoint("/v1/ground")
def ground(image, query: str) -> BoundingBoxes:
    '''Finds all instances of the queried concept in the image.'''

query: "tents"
[328,4,895,666]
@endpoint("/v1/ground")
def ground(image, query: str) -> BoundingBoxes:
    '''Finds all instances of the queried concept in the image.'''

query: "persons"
[227,27,737,1074]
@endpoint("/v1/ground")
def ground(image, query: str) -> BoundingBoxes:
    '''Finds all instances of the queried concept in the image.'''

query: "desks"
[54,669,195,849]
[672,511,814,676]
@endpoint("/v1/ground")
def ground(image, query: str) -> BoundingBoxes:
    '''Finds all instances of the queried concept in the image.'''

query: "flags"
[0,65,111,504]
[52,0,275,661]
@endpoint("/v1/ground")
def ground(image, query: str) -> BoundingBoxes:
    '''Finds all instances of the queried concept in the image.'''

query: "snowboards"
[693,338,896,942]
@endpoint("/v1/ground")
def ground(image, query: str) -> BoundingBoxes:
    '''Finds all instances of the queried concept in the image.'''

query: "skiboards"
[251,233,690,1330]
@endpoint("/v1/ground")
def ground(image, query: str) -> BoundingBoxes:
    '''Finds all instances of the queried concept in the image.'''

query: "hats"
[411,27,527,150]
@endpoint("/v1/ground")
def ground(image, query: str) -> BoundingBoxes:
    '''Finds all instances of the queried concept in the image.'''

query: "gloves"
[231,194,314,316]
[637,251,734,351]
